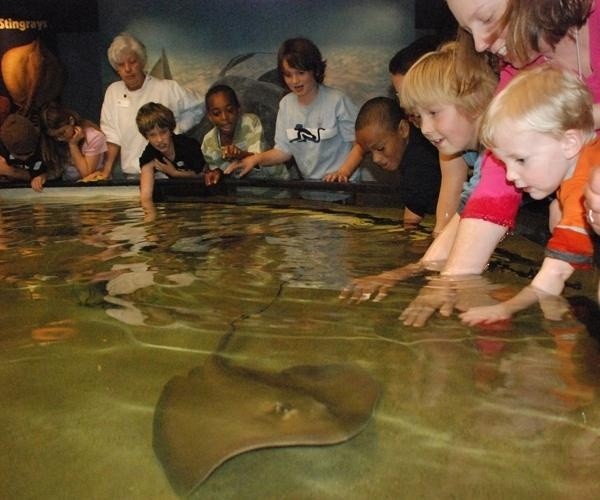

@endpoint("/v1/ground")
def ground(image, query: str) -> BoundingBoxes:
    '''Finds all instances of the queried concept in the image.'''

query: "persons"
[223,37,367,186]
[196,83,290,188]
[395,0,600,329]
[455,59,598,332]
[582,167,598,241]
[335,40,504,308]
[388,35,470,255]
[135,101,206,224]
[0,112,48,184]
[353,96,441,231]
[29,100,109,192]
[79,32,209,183]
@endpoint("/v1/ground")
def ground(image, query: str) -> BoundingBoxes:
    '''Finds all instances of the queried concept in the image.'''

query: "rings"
[588,209,594,225]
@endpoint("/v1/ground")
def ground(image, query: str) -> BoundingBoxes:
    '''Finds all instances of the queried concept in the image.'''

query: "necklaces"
[542,27,584,81]
